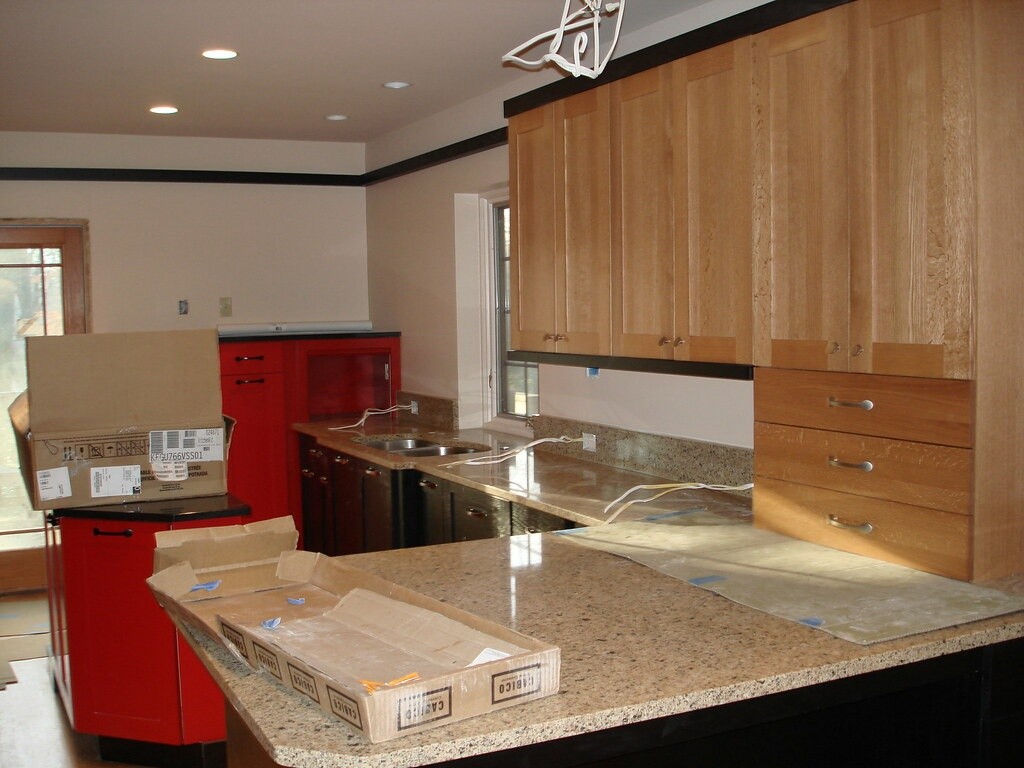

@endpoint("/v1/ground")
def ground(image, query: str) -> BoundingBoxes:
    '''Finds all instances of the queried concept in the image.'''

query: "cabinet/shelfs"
[420,471,566,546]
[216,329,402,551]
[752,0,1024,381]
[611,9,753,381]
[503,60,612,370]
[297,423,422,558]
[754,366,1023,584]
[43,491,251,768]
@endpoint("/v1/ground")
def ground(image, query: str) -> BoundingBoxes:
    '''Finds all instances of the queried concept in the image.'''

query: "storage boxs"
[145,514,561,745]
[8,329,238,511]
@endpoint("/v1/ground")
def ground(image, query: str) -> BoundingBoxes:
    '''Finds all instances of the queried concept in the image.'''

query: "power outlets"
[583,433,596,453]
[411,400,418,415]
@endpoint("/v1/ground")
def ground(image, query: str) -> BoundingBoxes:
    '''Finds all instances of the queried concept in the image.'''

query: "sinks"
[389,435,493,461]
[363,437,441,449]
